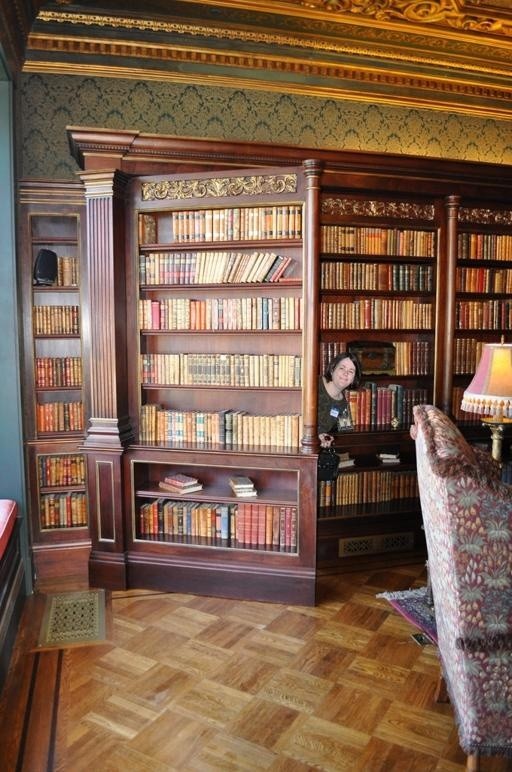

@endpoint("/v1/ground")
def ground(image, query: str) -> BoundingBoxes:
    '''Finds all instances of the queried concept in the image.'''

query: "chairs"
[414,406,512,772]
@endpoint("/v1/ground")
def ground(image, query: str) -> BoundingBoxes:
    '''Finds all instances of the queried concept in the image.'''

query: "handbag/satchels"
[318,447,340,481]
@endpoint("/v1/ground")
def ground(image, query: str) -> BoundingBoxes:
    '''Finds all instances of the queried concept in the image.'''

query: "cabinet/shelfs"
[15,159,512,608]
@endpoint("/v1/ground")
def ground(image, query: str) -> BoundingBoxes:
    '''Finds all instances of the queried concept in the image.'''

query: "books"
[32,256,90,532]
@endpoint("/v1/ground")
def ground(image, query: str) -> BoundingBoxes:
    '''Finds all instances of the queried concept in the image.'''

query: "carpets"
[375,587,438,647]
